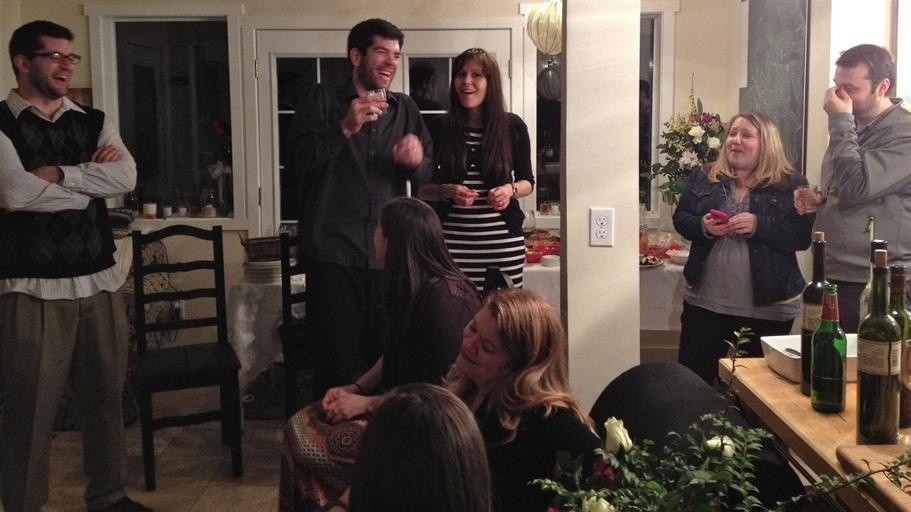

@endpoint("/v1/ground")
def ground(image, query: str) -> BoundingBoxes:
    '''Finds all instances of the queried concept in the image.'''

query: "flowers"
[208,117,232,179]
[647,71,727,217]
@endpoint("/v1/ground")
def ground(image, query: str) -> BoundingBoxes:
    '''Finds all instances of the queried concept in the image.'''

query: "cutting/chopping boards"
[836,441,911,511]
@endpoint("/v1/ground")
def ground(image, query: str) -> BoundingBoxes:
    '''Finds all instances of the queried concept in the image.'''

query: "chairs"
[132,225,242,492]
[280,232,312,425]
[52,230,180,431]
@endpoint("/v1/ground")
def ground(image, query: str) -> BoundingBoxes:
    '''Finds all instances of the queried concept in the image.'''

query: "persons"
[1,19,156,510]
[671,111,819,386]
[442,288,611,510]
[410,46,536,297]
[275,196,489,511]
[285,19,437,402]
[790,46,911,332]
[348,380,496,511]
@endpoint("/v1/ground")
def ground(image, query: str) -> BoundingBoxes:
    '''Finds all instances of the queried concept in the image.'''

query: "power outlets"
[589,208,615,247]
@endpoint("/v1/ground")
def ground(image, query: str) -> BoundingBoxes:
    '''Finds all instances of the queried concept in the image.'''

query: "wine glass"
[521,208,537,243]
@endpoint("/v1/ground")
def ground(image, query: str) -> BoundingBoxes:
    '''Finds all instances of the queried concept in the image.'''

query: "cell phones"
[710,209,729,224]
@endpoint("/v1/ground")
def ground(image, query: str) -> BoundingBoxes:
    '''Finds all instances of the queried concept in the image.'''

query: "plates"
[242,260,299,284]
[639,253,662,268]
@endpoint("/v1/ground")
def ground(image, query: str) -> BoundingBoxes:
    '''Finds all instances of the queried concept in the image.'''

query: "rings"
[332,408,339,415]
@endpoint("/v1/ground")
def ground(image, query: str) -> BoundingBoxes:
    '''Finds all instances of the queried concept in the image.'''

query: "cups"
[142,202,216,218]
[540,199,562,216]
[897,337,911,419]
[798,185,819,214]
[368,89,387,115]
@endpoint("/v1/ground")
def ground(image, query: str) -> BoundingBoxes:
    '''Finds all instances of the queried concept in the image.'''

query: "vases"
[217,174,227,206]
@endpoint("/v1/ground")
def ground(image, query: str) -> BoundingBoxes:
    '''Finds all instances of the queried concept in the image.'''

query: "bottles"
[811,280,847,414]
[798,229,831,392]
[859,236,890,324]
[856,252,901,451]
[883,262,911,337]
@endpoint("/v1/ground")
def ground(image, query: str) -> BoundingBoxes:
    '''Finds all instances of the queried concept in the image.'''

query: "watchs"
[512,182,520,198]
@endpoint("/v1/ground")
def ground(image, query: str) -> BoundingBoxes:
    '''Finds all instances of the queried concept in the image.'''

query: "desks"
[230,274,306,435]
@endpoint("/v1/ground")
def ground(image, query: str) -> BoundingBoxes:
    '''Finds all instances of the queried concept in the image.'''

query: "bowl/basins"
[665,250,689,264]
[238,232,288,262]
[525,249,562,266]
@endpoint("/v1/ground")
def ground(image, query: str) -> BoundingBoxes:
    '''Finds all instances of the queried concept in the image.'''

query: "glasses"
[30,51,81,64]
